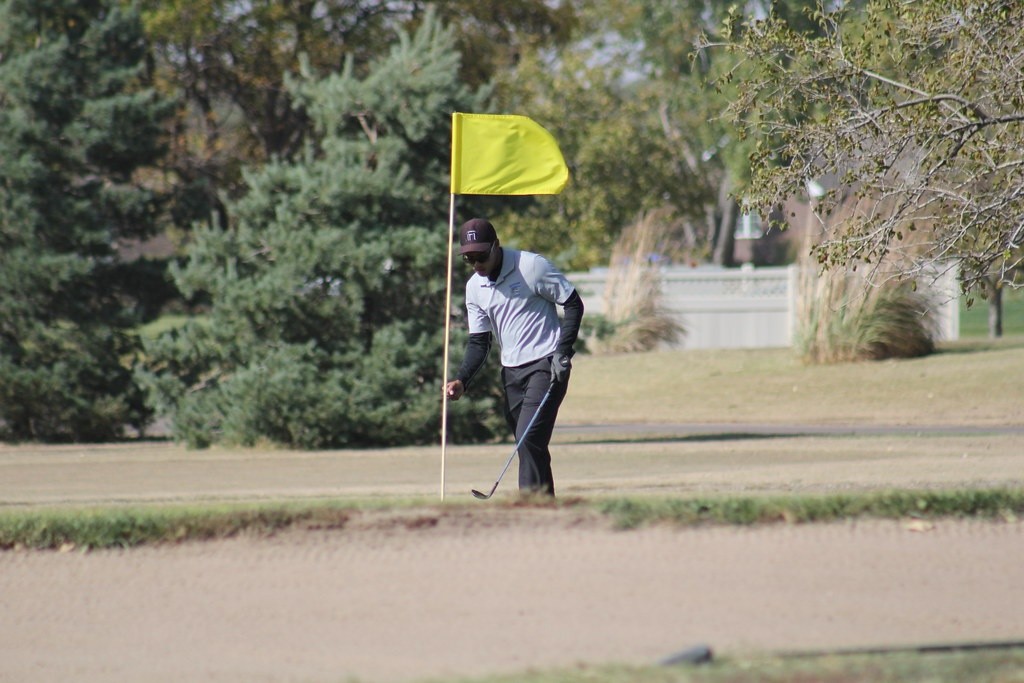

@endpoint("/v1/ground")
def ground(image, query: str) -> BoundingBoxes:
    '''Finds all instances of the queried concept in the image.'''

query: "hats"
[458,217,497,256]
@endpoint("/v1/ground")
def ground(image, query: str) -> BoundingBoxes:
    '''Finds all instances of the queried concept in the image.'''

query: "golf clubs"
[471,377,557,499]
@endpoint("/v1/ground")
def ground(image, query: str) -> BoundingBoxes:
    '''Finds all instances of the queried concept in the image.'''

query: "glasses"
[463,240,496,264]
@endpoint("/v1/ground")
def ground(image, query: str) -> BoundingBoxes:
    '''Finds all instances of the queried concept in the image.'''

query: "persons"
[441,220,584,500]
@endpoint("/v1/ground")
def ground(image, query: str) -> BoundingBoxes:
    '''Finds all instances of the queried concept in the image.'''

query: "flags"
[451,113,569,195]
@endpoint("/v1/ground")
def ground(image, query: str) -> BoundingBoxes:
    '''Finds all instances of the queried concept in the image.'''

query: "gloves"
[549,352,571,384]
